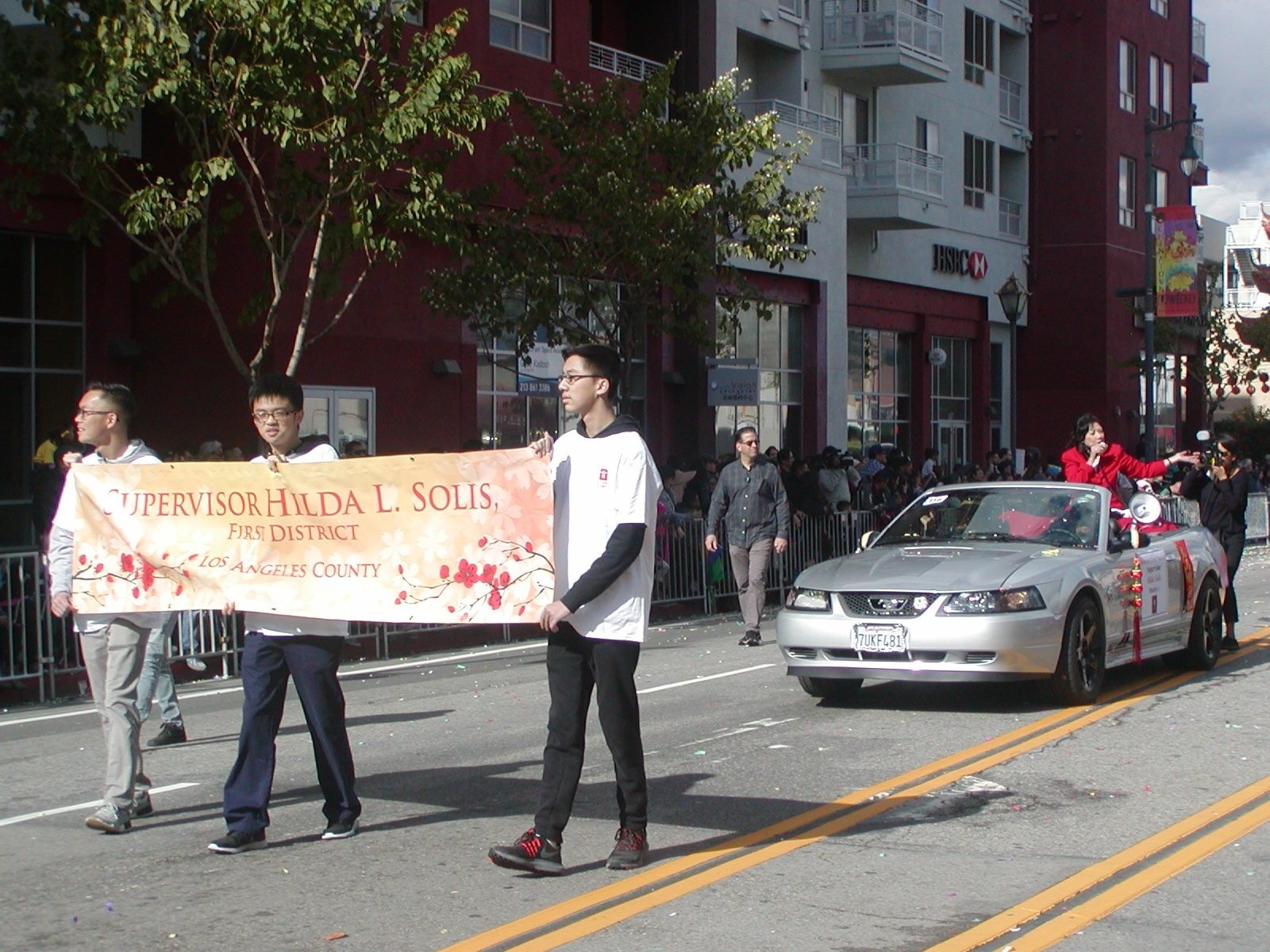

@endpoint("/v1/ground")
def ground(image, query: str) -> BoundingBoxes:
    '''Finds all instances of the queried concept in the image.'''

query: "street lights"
[994,270,1033,476]
[1142,114,1205,463]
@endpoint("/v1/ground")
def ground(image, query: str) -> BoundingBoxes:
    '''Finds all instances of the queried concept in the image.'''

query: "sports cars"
[775,480,1231,707]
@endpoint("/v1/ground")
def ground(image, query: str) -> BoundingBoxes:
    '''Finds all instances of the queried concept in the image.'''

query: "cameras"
[1197,429,1223,468]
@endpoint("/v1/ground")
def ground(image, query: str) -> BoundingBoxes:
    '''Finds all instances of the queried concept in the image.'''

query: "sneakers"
[147,722,186,746]
[608,826,649,868]
[83,803,133,832]
[207,827,266,853]
[738,630,762,646]
[127,792,155,817]
[322,816,358,839]
[489,829,564,872]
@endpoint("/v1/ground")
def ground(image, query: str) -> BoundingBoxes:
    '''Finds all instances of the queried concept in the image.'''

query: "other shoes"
[1219,635,1240,650]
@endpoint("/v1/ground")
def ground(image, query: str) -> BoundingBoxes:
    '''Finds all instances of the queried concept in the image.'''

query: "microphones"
[1097,441,1105,459]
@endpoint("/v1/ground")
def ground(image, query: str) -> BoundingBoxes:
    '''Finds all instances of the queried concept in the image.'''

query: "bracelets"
[1167,458,1172,465]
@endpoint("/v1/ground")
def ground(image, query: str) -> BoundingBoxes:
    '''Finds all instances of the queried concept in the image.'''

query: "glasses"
[74,407,119,422]
[252,407,300,422]
[558,373,605,385]
[738,439,760,447]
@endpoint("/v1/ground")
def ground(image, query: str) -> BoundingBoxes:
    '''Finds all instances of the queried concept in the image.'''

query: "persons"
[1117,433,1270,499]
[28,415,246,692]
[488,344,664,877]
[1180,432,1248,651]
[1060,414,1201,534]
[206,372,363,854]
[651,440,1063,616]
[46,383,188,835]
[705,427,790,647]
[343,441,366,458]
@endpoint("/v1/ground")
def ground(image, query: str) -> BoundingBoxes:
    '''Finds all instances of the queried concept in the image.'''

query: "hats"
[868,444,889,454]
[900,455,915,465]
[822,446,841,453]
